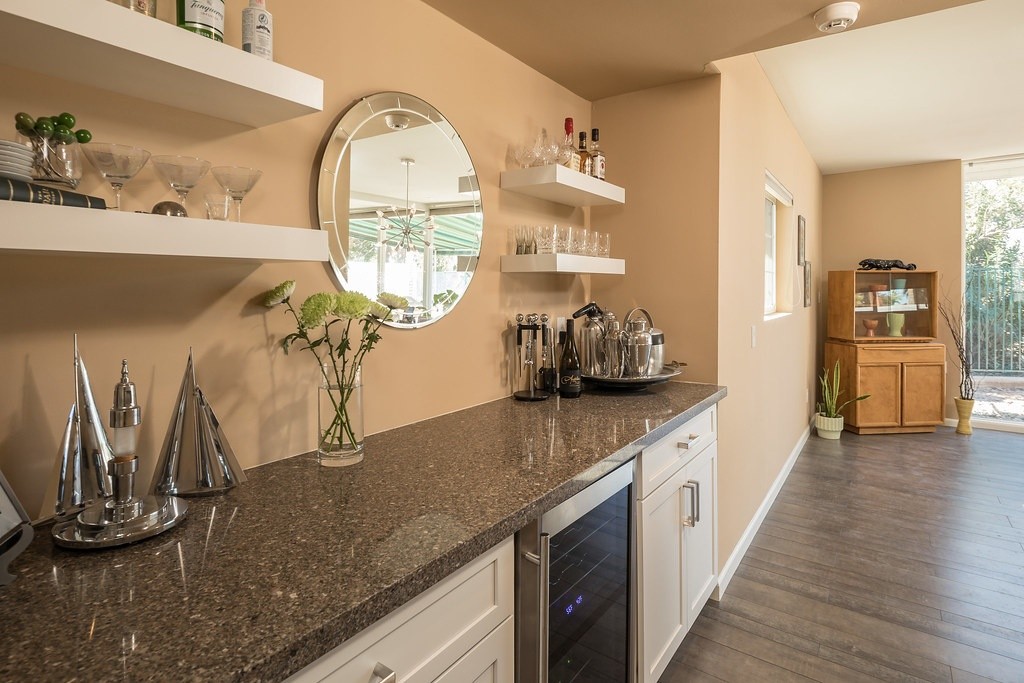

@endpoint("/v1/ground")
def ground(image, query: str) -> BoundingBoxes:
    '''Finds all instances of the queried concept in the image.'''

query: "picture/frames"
[798,214,805,266]
[804,260,812,307]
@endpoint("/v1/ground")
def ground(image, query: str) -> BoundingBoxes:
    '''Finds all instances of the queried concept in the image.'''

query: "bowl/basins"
[869,279,906,295]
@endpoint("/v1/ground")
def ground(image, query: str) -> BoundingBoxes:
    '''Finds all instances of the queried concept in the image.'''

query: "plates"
[0,140,34,183]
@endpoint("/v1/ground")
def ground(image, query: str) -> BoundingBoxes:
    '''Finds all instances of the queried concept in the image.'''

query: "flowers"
[259,278,411,452]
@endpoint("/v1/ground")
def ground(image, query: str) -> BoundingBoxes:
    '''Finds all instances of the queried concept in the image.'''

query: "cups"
[556,227,571,253]
[587,231,599,257]
[571,228,587,255]
[534,225,557,254]
[599,233,610,258]
[206,194,232,221]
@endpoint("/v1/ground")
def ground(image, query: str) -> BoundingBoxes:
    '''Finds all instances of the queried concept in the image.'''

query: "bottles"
[578,132,594,177]
[572,302,620,378]
[555,319,581,398]
[242,0,273,62]
[589,129,606,181]
[560,118,580,172]
[176,0,225,43]
[151,155,211,207]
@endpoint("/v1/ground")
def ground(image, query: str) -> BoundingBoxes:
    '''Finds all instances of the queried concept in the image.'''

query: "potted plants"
[813,355,873,440]
[934,278,995,433]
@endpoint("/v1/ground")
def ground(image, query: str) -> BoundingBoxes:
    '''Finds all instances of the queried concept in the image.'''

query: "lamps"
[372,158,439,256]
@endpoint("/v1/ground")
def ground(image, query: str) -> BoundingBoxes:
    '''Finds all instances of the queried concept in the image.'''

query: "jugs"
[620,317,653,377]
[623,307,664,375]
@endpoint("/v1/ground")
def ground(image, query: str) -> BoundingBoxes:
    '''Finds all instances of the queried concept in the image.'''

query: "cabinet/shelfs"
[820,342,948,434]
[499,164,628,274]
[637,403,721,683]
[0,0,332,265]
[283,532,520,683]
[826,269,940,342]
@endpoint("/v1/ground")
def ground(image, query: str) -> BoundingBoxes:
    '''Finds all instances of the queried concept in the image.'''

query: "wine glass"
[886,313,905,336]
[515,226,533,255]
[514,134,559,168]
[80,143,151,212]
[211,167,264,223]
[863,319,878,336]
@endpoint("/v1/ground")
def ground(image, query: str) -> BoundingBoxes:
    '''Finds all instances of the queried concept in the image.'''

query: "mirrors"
[314,89,484,333]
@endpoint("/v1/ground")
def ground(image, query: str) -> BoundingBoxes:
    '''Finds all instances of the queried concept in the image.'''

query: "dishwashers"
[516,456,637,683]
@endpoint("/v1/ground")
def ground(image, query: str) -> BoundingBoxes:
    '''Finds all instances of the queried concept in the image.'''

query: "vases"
[316,356,364,468]
